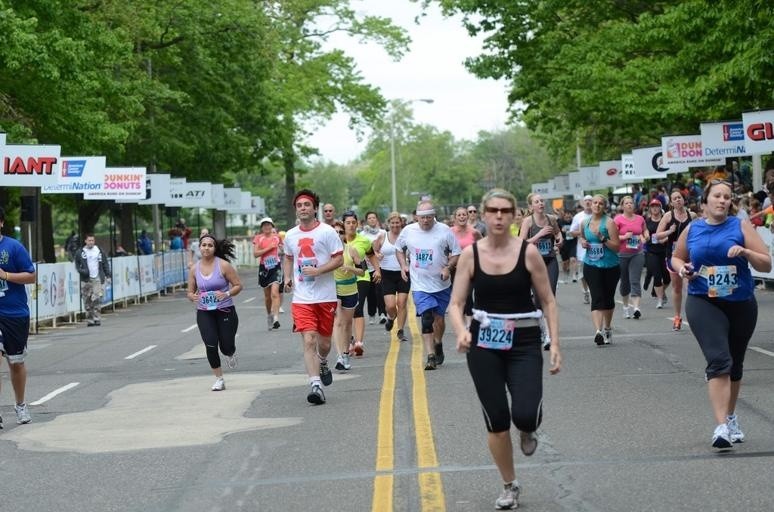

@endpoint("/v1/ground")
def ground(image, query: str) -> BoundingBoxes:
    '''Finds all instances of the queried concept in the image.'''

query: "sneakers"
[306,312,447,373]
[518,427,540,458]
[711,423,735,449]
[266,314,281,332]
[211,375,226,391]
[87,320,102,327]
[14,401,34,424]
[223,353,240,370]
[306,381,327,405]
[315,356,335,387]
[727,414,746,444]
[493,480,524,511]
[538,290,695,353]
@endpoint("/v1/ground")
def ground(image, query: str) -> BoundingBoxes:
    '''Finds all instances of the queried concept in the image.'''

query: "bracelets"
[224,288,230,298]
[6,271,9,281]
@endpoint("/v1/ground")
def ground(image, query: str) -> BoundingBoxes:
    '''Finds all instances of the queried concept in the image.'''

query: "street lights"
[391,97,435,218]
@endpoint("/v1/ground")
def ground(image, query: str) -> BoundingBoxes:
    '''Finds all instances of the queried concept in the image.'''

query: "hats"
[260,217,276,228]
[648,199,663,207]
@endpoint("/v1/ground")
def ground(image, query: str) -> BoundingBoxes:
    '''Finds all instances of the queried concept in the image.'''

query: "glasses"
[710,179,733,187]
[484,206,516,214]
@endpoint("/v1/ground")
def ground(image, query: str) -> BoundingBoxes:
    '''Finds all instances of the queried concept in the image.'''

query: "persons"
[282,187,346,405]
[170,218,192,249]
[74,232,112,327]
[251,160,772,373]
[135,230,153,255]
[445,188,562,511]
[668,176,771,449]
[184,234,244,392]
[0,209,37,431]
[162,230,184,251]
[186,228,210,303]
[107,238,126,258]
